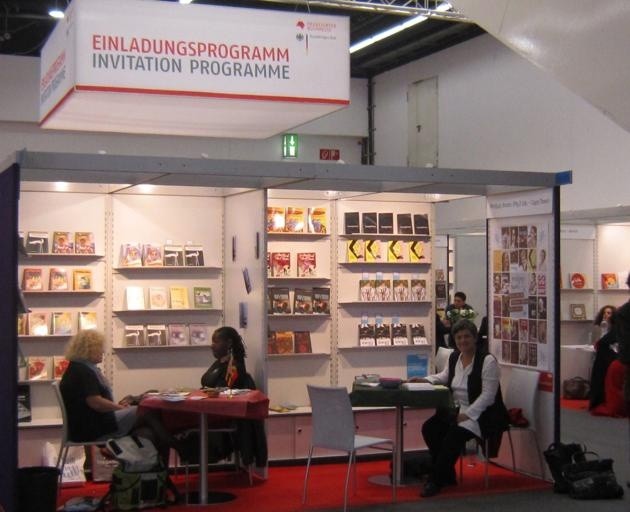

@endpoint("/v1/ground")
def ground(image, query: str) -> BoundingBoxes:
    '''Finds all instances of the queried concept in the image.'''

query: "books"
[407,352,429,379]
[18,230,96,379]
[435,269,446,322]
[353,381,384,391]
[571,303,586,321]
[123,242,213,345]
[601,273,619,289]
[266,207,430,353]
[402,382,436,391]
[569,271,588,289]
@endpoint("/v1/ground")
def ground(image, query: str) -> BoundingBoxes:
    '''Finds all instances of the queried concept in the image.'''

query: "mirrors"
[433,198,488,374]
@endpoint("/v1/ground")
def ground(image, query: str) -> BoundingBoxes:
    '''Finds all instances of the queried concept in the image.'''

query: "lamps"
[350,2,451,54]
[179,0,192,5]
[48,0,65,18]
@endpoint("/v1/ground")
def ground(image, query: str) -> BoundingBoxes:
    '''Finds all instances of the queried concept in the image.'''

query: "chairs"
[302,384,396,512]
[52,381,105,494]
[436,346,455,373]
[460,366,546,487]
[174,374,255,503]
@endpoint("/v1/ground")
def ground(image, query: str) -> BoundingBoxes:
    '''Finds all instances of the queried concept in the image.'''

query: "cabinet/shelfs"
[336,165,433,457]
[16,152,106,476]
[107,155,264,471]
[560,289,630,323]
[265,162,336,462]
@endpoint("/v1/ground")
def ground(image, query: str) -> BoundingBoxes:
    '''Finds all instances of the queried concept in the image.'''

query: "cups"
[413,272,421,281]
[376,271,382,280]
[363,273,369,280]
[207,388,219,398]
[393,271,400,280]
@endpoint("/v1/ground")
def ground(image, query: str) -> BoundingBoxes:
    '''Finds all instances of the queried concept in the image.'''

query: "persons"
[168,326,248,466]
[594,272,629,410]
[407,320,509,497]
[60,329,169,470]
[478,304,488,353]
[494,226,546,367]
[436,312,451,354]
[446,292,473,348]
[595,305,616,344]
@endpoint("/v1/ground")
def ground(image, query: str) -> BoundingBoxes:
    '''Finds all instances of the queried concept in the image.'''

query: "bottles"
[375,313,384,328]
[226,388,232,400]
[391,313,400,323]
[361,312,369,328]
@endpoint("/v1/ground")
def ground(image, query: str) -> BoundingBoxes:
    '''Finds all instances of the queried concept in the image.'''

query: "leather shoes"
[421,482,439,496]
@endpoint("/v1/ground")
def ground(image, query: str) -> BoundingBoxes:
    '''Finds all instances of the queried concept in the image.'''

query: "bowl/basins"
[200,388,208,393]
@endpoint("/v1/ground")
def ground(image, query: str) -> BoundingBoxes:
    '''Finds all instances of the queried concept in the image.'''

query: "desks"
[352,383,449,487]
[140,388,269,505]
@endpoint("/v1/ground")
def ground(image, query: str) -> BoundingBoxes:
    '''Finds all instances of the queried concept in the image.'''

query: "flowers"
[447,309,478,325]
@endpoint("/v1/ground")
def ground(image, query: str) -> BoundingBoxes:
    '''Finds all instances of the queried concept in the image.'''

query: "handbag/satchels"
[105,434,171,511]
[563,376,592,400]
[562,451,624,499]
[543,442,586,493]
